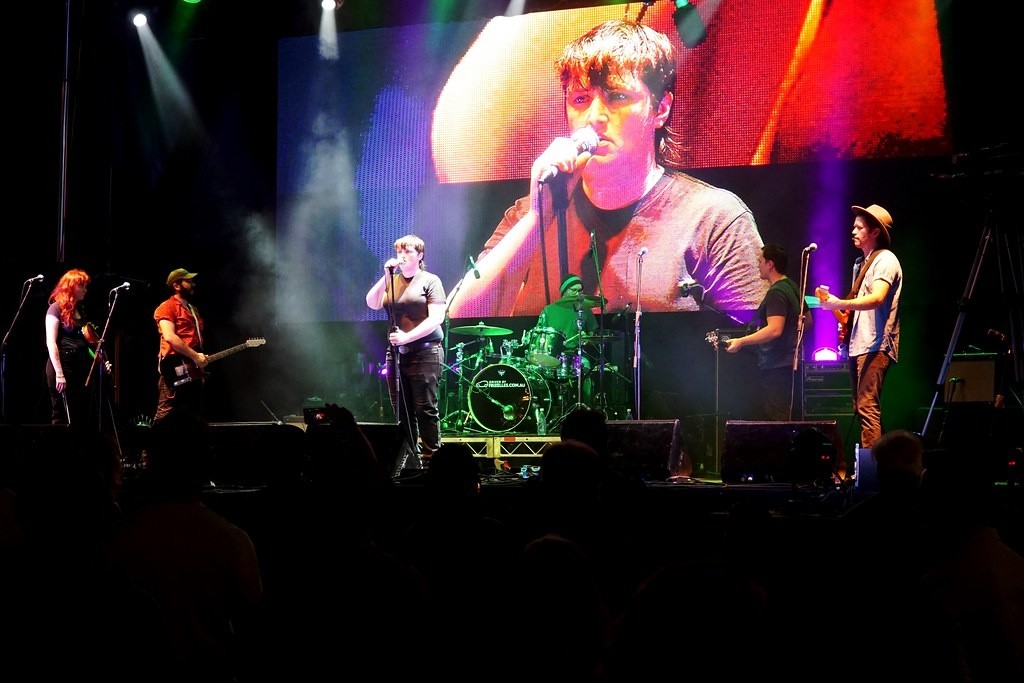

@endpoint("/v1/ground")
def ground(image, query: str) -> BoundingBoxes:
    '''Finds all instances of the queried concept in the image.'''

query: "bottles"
[537,408,546,436]
[626,408,633,421]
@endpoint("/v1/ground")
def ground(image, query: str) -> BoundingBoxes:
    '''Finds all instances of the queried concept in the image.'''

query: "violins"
[81,322,120,390]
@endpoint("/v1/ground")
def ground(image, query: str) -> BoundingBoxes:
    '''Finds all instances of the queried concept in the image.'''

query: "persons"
[723,245,806,421]
[366,234,447,471]
[0,407,1024,683]
[45,267,95,424]
[154,267,208,424]
[530,273,602,404]
[446,20,777,317]
[819,204,903,450]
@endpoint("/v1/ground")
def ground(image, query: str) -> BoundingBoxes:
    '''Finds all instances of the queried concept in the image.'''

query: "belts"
[387,342,440,354]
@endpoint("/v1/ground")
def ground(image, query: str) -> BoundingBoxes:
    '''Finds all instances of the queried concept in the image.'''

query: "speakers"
[305,421,421,483]
[605,418,692,482]
[943,352,1009,405]
[858,448,945,494]
[716,329,804,475]
[0,422,69,518]
[206,424,306,489]
[720,420,846,484]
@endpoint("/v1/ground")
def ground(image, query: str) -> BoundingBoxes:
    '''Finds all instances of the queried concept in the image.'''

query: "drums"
[541,349,582,381]
[525,327,567,367]
[581,329,622,339]
[467,364,552,433]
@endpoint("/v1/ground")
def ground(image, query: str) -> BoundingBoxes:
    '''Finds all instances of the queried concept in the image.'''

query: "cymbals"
[449,325,514,336]
[555,295,608,309]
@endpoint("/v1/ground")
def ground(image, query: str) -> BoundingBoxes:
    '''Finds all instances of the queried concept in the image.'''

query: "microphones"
[112,282,131,291]
[573,340,589,353]
[489,339,494,354]
[949,378,965,383]
[590,230,594,252]
[612,303,631,324]
[637,247,648,257]
[386,259,404,269]
[468,254,480,279]
[805,243,818,254]
[26,275,44,283]
[503,404,517,420]
[539,126,600,184]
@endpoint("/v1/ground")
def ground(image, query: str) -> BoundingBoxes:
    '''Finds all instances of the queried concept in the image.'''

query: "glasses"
[568,287,583,294]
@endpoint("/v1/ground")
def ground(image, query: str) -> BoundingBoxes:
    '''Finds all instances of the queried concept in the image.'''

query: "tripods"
[917,186,1024,444]
[548,244,610,434]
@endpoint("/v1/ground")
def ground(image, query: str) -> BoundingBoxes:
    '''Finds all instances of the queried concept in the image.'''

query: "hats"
[166,268,198,288]
[559,274,583,296]
[851,204,894,246]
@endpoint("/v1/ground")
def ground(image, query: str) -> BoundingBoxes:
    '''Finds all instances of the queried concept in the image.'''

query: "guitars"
[159,336,269,397]
[714,321,760,353]
[814,285,856,348]
